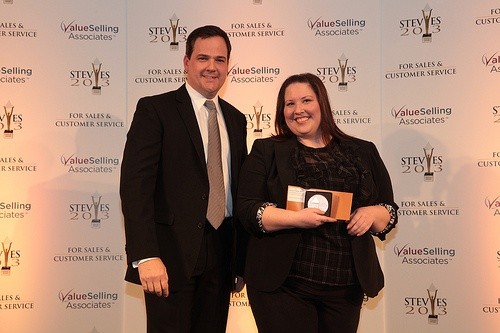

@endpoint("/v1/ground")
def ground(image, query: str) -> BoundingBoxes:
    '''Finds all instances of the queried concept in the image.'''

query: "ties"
[203,100,225,230]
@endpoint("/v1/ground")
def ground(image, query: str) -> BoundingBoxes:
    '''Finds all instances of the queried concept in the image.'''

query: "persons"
[120,25,249,333]
[242,72,399,333]
